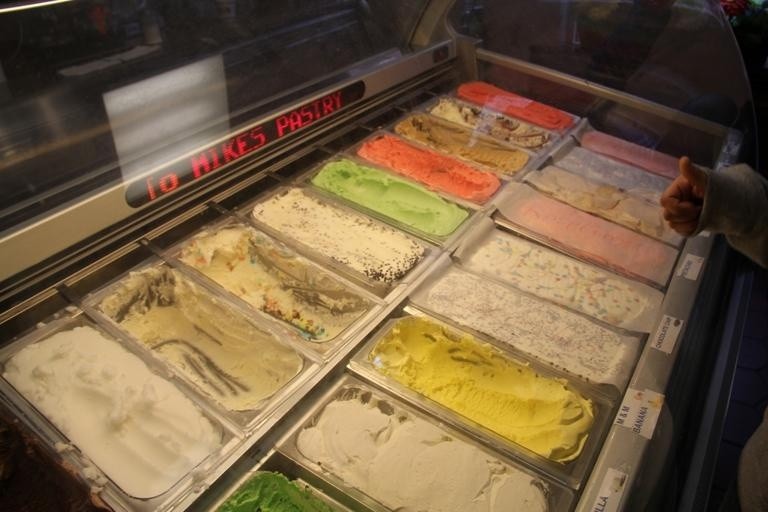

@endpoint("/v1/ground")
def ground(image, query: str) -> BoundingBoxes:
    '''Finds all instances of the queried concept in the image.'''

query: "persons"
[660,156,768,512]
[564,3,757,175]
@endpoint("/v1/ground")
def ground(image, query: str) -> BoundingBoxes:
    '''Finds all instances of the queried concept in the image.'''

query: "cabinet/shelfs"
[0,0,753,511]
